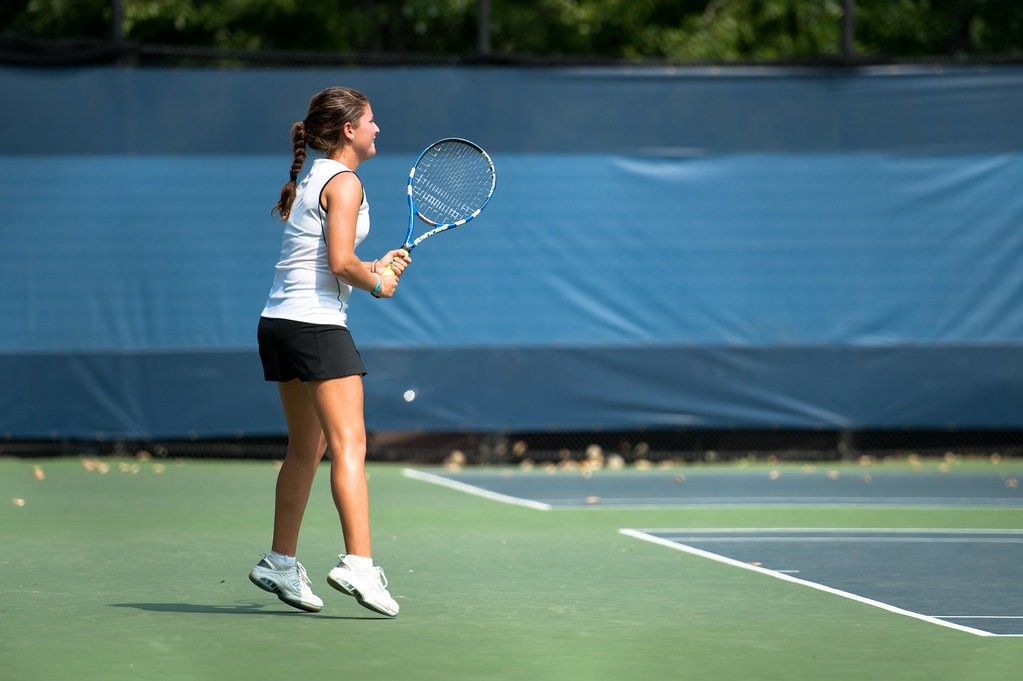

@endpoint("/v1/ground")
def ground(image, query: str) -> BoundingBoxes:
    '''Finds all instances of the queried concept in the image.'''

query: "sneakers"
[327,554,399,616]
[249,552,324,612]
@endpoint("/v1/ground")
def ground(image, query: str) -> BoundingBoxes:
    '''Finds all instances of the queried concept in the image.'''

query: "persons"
[248,84,411,619]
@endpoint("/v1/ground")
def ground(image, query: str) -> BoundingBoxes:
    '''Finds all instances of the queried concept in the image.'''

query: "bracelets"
[370,259,378,272]
[369,273,380,295]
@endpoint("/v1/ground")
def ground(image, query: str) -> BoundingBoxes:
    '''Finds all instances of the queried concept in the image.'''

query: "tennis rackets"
[369,136,497,300]
